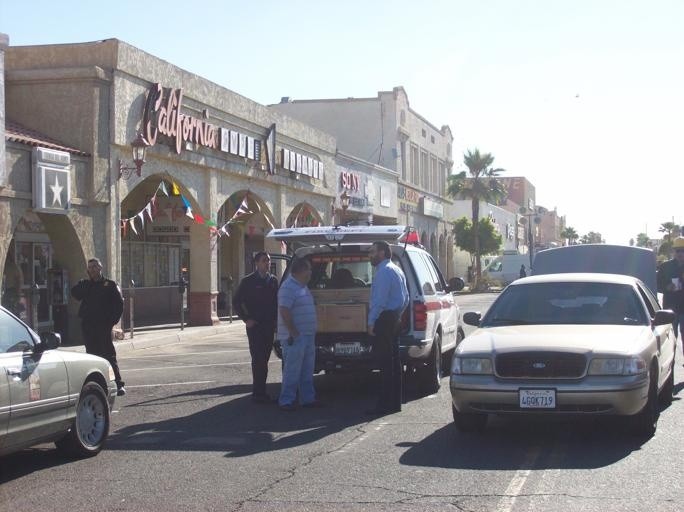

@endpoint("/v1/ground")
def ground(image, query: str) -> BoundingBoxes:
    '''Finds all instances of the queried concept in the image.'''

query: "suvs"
[252,220,465,399]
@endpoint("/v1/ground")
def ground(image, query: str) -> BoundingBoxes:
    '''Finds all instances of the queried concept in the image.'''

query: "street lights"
[518,203,545,277]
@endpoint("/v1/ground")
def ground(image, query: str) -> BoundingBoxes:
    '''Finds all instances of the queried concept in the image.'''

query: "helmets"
[672,236,684,248]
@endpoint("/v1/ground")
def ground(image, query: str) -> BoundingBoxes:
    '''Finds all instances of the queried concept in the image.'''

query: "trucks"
[471,252,531,289]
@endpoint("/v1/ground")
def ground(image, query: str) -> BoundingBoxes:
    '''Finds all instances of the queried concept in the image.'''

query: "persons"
[276,257,325,411]
[519,264,526,278]
[232,252,279,404]
[364,240,411,415]
[3,254,23,318]
[71,258,127,397]
[657,236,684,369]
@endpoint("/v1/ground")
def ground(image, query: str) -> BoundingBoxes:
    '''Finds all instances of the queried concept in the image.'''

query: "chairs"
[332,268,353,288]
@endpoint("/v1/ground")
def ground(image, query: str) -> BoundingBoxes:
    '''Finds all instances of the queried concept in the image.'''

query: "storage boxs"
[322,302,368,334]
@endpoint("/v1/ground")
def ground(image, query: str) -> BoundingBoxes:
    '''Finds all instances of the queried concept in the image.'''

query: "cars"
[0,302,119,462]
[449,244,679,437]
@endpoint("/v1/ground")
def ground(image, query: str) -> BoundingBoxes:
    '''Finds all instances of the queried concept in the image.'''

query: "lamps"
[331,191,351,216]
[118,127,151,181]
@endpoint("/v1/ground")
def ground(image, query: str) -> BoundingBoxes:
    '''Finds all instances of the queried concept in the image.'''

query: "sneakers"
[118,385,126,395]
[366,408,401,414]
[252,395,327,411]
